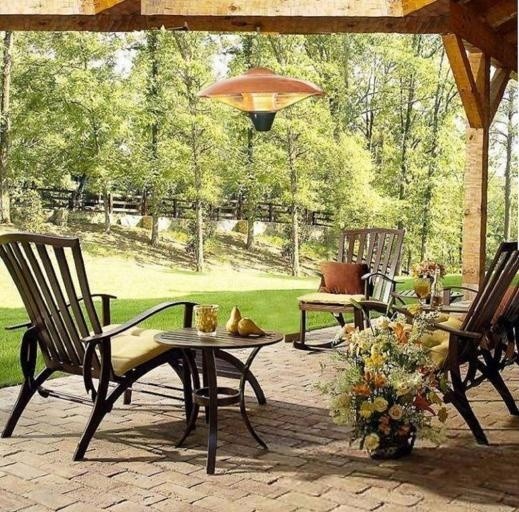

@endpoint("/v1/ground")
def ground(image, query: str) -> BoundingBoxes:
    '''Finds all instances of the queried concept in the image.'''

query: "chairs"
[0,232,200,461]
[354,239,518,457]
[292,225,408,355]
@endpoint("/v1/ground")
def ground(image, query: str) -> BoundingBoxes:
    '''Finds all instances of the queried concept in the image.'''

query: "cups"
[195,305,219,335]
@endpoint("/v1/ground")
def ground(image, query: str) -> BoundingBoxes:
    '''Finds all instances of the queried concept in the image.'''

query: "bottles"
[431,267,444,309]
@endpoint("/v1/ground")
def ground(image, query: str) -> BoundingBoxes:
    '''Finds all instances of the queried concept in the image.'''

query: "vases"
[368,434,416,460]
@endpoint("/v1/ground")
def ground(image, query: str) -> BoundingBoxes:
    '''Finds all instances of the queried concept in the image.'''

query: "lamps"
[196,21,325,131]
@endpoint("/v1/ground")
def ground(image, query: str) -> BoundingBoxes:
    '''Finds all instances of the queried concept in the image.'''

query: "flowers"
[314,303,452,456]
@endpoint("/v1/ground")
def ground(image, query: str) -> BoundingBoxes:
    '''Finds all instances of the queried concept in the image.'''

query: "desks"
[152,330,284,474]
[391,286,466,308]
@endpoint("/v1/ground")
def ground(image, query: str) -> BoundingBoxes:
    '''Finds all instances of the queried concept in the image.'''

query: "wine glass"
[413,278,431,308]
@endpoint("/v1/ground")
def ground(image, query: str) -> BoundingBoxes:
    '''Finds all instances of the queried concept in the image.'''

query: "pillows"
[318,259,373,297]
